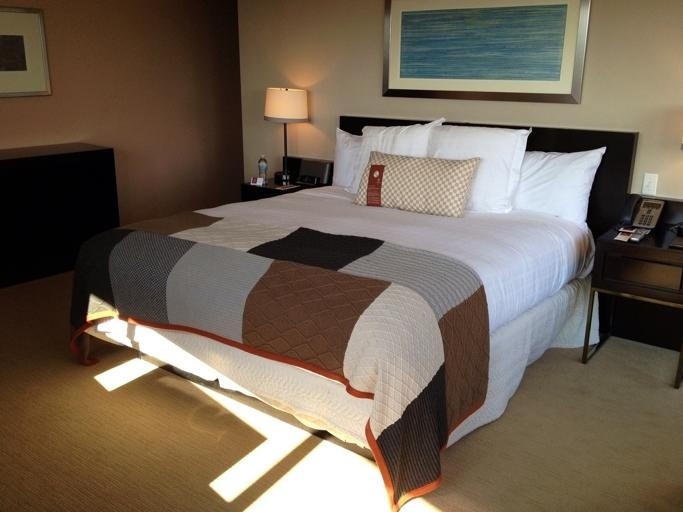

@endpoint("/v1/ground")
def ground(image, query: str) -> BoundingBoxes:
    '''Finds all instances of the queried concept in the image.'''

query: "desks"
[0,142,121,288]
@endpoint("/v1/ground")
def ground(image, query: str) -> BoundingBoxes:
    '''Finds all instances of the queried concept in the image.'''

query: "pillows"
[331,118,608,235]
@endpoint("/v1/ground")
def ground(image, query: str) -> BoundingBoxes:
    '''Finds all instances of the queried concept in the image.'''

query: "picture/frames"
[382,0,590,101]
[1,5,53,98]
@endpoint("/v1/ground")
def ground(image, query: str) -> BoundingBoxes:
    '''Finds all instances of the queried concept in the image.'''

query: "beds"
[75,114,640,462]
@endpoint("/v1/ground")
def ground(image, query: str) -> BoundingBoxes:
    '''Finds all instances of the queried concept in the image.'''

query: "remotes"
[631,230,645,243]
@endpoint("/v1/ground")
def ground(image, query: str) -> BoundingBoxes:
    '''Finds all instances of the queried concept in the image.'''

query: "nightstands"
[241,175,325,202]
[581,195,683,389]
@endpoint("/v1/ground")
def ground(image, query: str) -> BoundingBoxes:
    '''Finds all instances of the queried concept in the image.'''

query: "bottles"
[258,155,268,185]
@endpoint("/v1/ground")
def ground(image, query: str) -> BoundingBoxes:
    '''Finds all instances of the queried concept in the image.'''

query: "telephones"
[620,194,667,229]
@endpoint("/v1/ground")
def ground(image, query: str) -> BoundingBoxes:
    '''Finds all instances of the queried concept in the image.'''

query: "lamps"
[263,87,309,180]
